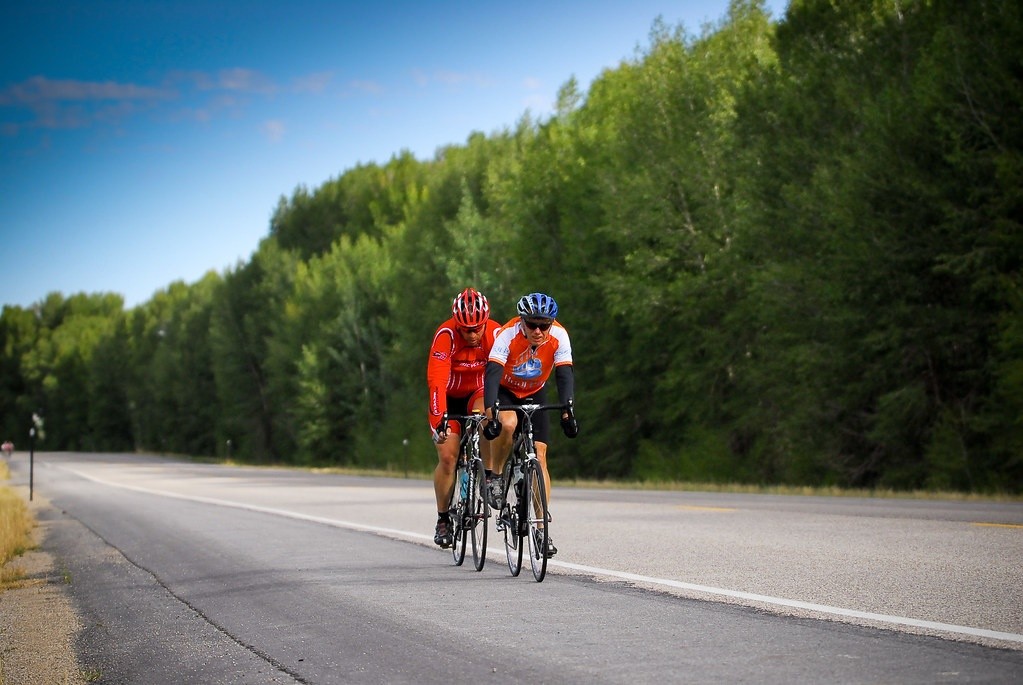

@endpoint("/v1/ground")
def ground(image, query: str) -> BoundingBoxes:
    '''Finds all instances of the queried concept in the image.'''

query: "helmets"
[452,287,490,327]
[516,293,558,318]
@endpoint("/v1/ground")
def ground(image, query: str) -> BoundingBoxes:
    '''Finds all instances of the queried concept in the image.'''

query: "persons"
[483,294,579,559]
[428,285,505,549]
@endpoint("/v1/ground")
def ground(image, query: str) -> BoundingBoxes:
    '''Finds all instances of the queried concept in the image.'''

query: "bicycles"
[493,396,575,583]
[440,410,493,572]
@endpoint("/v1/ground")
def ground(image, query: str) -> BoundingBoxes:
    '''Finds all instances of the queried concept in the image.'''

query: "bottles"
[513,458,525,494]
[460,468,470,500]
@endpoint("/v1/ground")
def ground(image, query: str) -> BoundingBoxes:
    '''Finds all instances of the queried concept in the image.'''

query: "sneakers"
[486,475,505,509]
[535,528,558,554]
[478,478,492,499]
[434,516,454,547]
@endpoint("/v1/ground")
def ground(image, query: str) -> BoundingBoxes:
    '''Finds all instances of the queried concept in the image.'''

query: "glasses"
[523,317,552,331]
[461,325,482,333]
